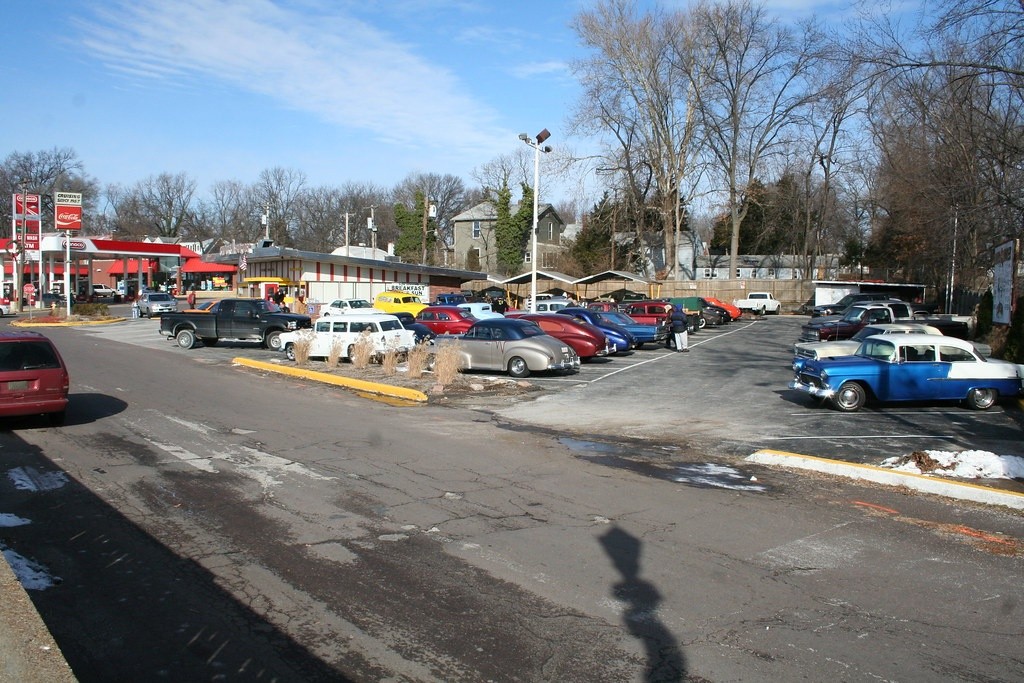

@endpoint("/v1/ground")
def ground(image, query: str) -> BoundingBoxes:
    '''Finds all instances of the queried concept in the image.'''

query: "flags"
[238,251,248,270]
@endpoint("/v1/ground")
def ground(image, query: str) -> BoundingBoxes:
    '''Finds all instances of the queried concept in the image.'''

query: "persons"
[483,293,509,314]
[188,289,195,309]
[266,291,291,313]
[131,299,138,319]
[663,304,690,352]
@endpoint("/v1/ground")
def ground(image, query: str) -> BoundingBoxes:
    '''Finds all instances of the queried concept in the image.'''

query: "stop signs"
[23,283,36,295]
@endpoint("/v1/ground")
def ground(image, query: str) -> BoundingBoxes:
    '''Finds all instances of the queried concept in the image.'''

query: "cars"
[318,298,385,317]
[386,305,484,345]
[34,293,74,308]
[279,312,416,364]
[92,283,116,298]
[423,289,741,335]
[555,307,638,355]
[181,298,235,313]
[506,312,617,363]
[136,291,178,318]
[600,312,671,349]
[788,292,1024,412]
[424,317,581,378]
[0,328,70,425]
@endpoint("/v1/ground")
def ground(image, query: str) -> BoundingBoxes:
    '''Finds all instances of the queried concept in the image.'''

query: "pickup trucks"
[159,297,312,352]
[731,291,782,316]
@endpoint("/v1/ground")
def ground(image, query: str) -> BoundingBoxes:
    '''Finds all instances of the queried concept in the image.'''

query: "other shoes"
[678,350,681,353]
[682,349,690,352]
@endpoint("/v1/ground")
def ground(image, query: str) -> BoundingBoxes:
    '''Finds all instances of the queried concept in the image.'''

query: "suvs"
[372,289,430,317]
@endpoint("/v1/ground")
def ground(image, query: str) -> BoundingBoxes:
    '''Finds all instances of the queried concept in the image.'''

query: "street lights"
[517,128,553,314]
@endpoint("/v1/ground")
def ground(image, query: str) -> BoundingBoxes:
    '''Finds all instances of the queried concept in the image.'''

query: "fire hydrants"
[50,302,57,311]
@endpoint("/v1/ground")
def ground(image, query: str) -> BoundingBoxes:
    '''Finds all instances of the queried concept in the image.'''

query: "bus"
[0,279,18,318]
